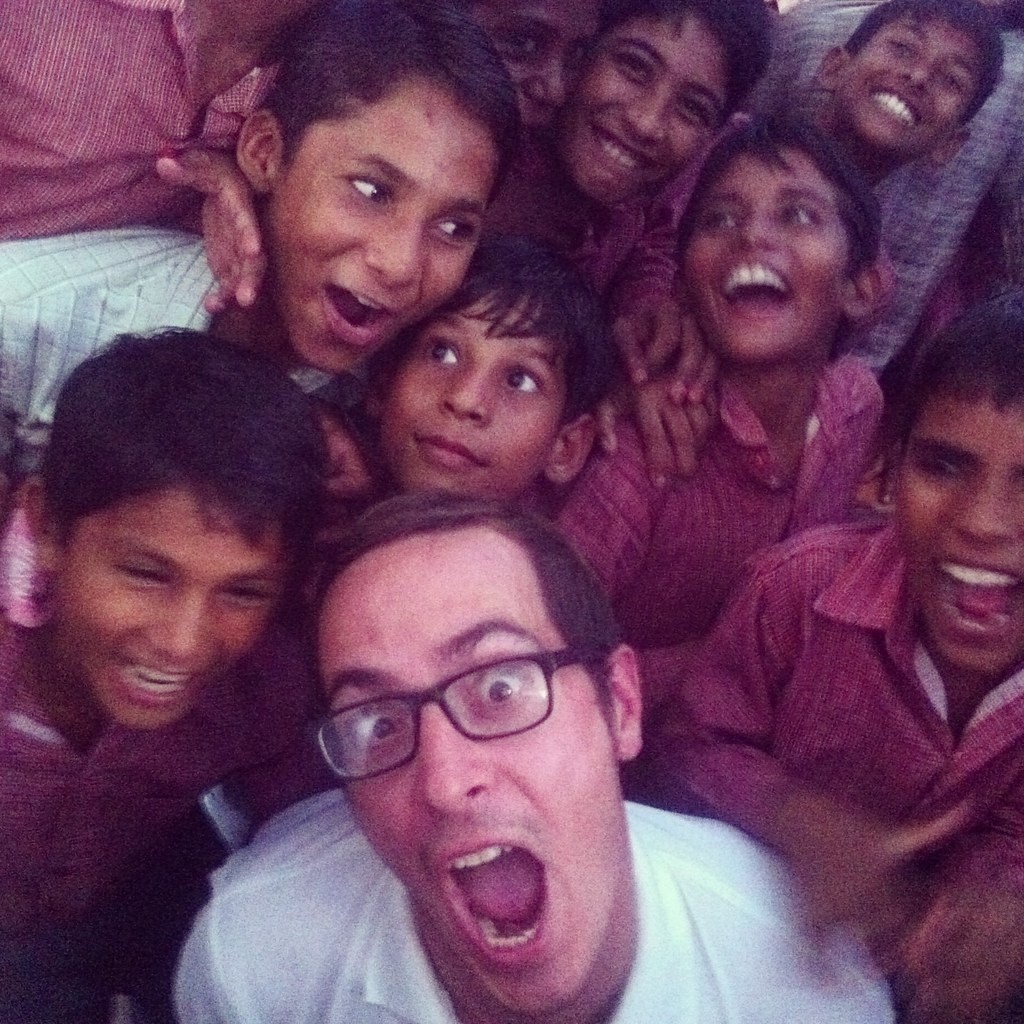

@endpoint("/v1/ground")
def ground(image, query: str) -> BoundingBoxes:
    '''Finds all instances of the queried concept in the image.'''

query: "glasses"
[318,645,584,780]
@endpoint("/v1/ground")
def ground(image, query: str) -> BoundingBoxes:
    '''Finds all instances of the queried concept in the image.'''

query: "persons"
[2,0,1023,1024]
[171,494,898,1024]
